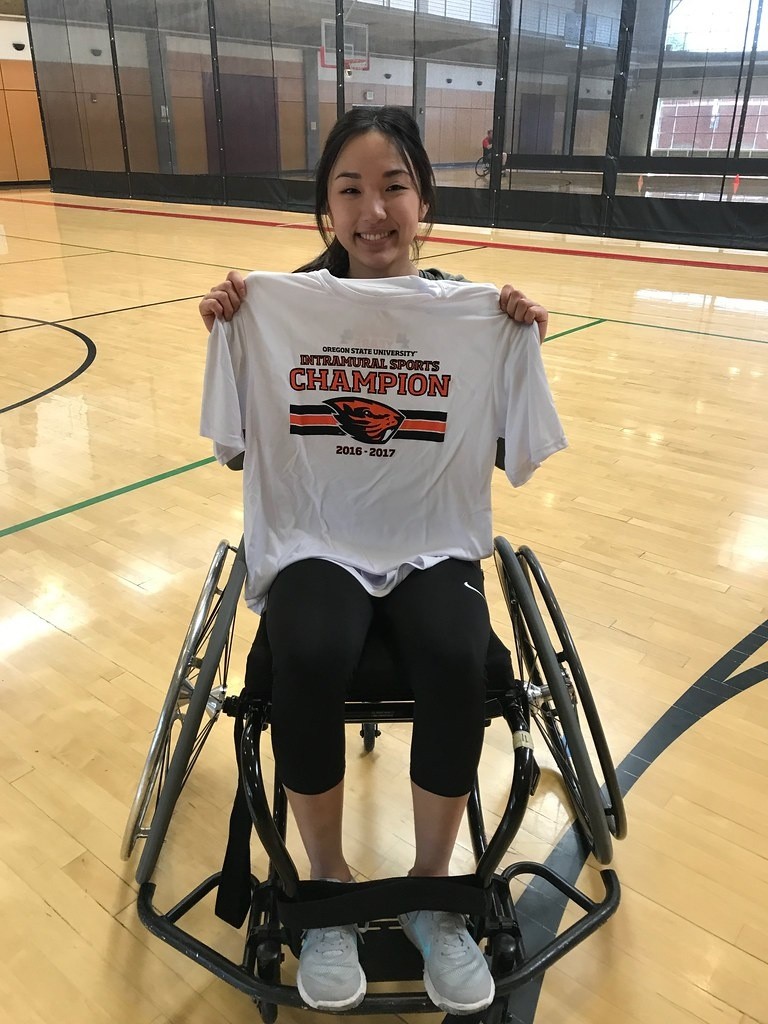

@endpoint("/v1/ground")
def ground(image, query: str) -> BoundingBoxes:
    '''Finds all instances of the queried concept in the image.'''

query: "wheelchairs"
[124,535,627,1023]
[475,157,505,176]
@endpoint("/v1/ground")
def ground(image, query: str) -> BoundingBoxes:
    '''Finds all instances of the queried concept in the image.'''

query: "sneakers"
[296,878,370,1012]
[398,869,497,1017]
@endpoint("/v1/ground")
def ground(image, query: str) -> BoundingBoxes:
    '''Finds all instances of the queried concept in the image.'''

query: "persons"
[199,105,550,1016]
[483,128,508,177]
